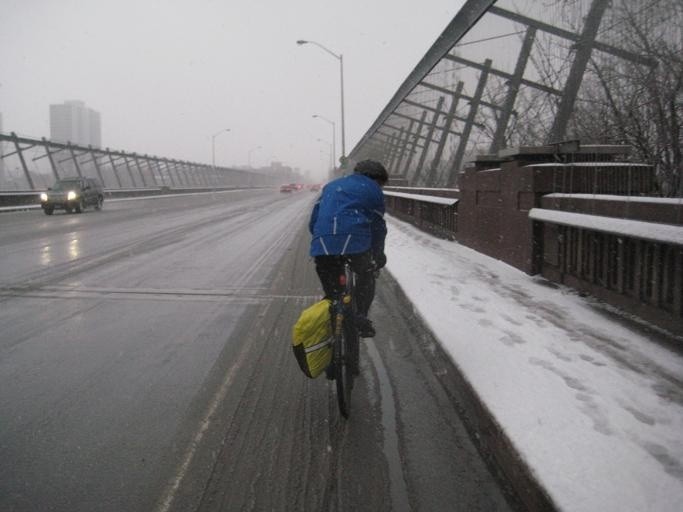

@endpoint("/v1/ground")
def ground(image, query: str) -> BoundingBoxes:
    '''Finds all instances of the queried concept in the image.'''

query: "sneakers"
[353,312,376,336]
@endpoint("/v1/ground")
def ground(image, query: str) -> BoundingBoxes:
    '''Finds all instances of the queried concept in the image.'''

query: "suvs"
[39,177,104,216]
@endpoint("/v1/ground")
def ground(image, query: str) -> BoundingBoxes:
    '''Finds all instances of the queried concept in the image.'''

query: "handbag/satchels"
[292,296,334,378]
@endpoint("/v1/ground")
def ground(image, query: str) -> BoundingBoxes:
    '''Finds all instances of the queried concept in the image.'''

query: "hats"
[355,159,388,182]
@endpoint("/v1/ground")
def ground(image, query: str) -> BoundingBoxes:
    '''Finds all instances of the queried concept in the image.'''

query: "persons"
[306,160,389,381]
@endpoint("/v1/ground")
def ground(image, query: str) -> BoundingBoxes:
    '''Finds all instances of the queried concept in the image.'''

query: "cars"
[278,182,323,192]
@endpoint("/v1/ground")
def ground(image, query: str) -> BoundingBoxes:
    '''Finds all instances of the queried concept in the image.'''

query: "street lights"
[247,145,292,186]
[295,39,346,161]
[210,129,231,190]
[316,138,332,176]
[312,115,335,171]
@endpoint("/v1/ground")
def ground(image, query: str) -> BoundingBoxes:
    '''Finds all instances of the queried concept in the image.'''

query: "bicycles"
[326,253,381,419]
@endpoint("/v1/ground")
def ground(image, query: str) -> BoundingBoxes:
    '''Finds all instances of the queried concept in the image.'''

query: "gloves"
[376,252,387,267]
